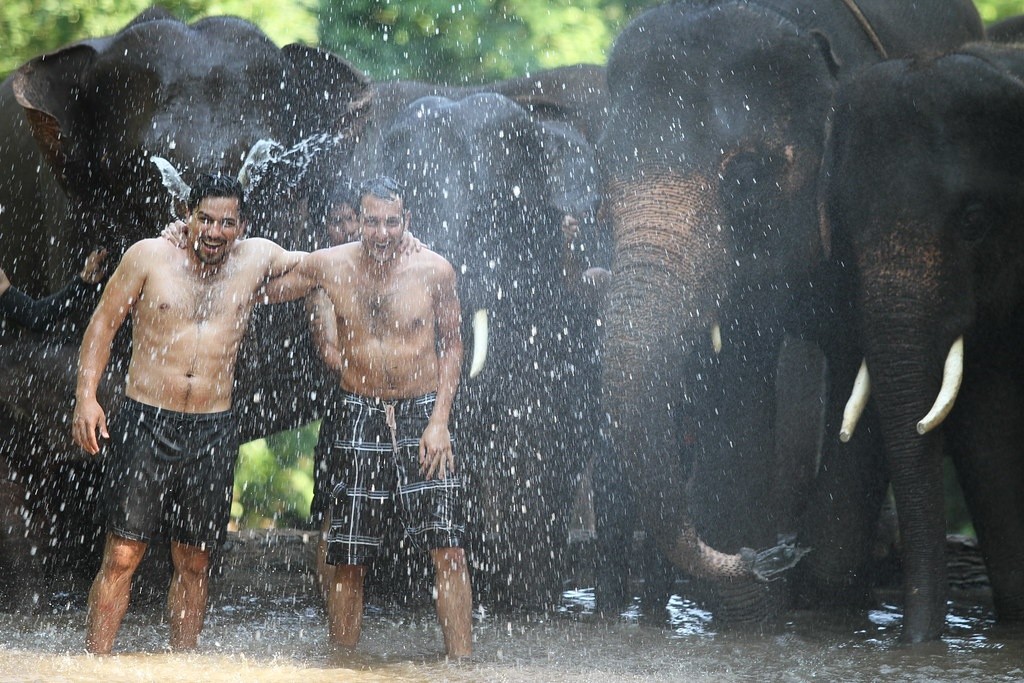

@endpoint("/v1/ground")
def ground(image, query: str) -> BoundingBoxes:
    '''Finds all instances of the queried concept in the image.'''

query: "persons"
[159,176,481,667]
[69,172,344,652]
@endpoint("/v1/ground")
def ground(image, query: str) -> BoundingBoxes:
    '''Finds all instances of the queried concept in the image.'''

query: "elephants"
[4,0,1024,654]
